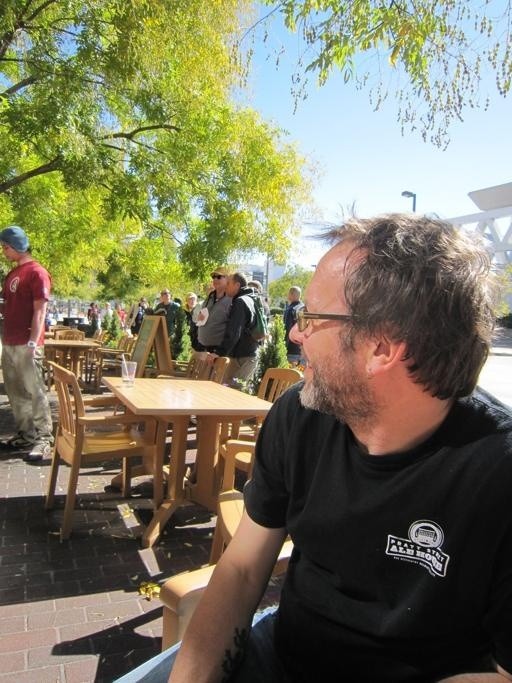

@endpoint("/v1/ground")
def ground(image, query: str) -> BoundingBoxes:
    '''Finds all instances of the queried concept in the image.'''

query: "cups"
[121,361,137,387]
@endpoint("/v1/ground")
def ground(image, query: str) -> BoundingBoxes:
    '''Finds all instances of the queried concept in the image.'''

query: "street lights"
[402,190,417,212]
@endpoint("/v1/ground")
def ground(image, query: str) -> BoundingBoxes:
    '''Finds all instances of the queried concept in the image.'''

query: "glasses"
[296,305,361,332]
[212,275,225,279]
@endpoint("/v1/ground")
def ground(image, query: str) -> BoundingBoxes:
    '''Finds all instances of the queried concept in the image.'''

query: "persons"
[0,226,55,461]
[87,303,99,324]
[205,272,261,383]
[129,297,150,338]
[284,286,305,363]
[154,288,178,337]
[167,209,512,683]
[99,301,113,322]
[247,280,271,325]
[182,292,198,352]
[196,267,233,354]
[115,304,127,330]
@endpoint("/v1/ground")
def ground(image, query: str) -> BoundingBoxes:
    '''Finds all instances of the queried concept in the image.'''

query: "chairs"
[159,540,296,660]
[209,489,295,585]
[85,328,140,380]
[55,331,84,384]
[216,368,305,504]
[158,352,232,388]
[45,361,149,541]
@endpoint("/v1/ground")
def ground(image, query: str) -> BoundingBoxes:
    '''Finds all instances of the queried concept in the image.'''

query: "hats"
[186,292,197,302]
[0,226,29,252]
[210,266,234,277]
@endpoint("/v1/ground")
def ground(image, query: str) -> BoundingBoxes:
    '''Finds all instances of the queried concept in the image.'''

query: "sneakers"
[28,440,50,459]
[0,433,32,447]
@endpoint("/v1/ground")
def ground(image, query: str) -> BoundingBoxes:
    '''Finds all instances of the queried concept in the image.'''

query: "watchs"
[26,339,41,351]
